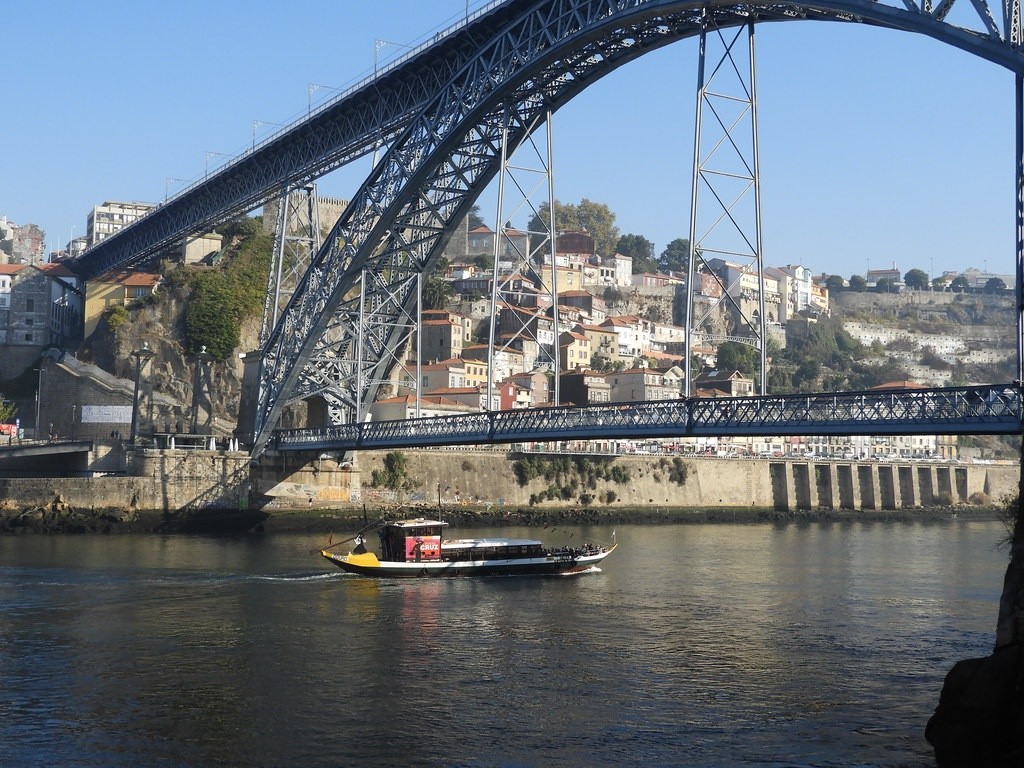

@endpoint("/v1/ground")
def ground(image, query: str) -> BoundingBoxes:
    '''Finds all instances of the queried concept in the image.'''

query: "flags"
[610,530,614,538]
[329,532,332,545]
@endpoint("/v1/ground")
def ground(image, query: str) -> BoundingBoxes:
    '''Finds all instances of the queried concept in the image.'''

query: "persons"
[544,543,606,556]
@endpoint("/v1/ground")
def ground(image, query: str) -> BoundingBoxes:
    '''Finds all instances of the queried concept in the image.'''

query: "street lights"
[70,226,76,256]
[34,368,45,440]
[72,405,76,445]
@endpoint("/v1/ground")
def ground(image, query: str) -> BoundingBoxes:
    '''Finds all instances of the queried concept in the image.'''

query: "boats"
[310,483,619,579]
